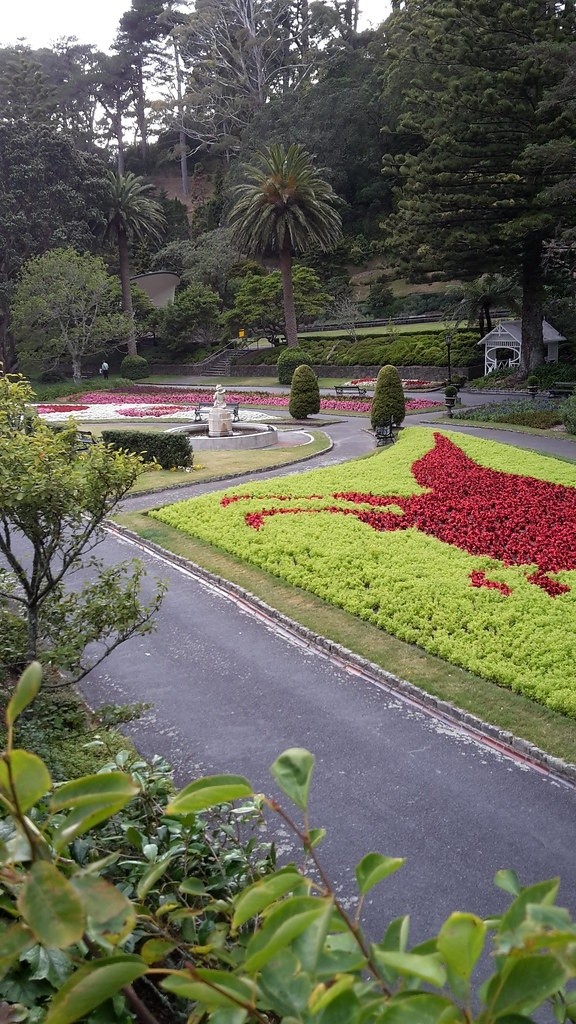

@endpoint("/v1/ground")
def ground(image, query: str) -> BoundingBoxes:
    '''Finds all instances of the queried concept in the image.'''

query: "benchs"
[81,370,94,379]
[76,430,104,444]
[546,381,576,398]
[375,415,395,447]
[193,402,241,422]
[334,386,367,396]
[281,337,307,345]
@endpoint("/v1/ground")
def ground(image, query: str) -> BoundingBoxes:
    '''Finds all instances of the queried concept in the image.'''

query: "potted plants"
[527,376,540,392]
[451,374,466,392]
[444,386,458,405]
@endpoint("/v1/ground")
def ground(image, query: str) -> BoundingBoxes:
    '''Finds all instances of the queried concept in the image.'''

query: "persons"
[101,360,109,380]
[213,384,227,409]
